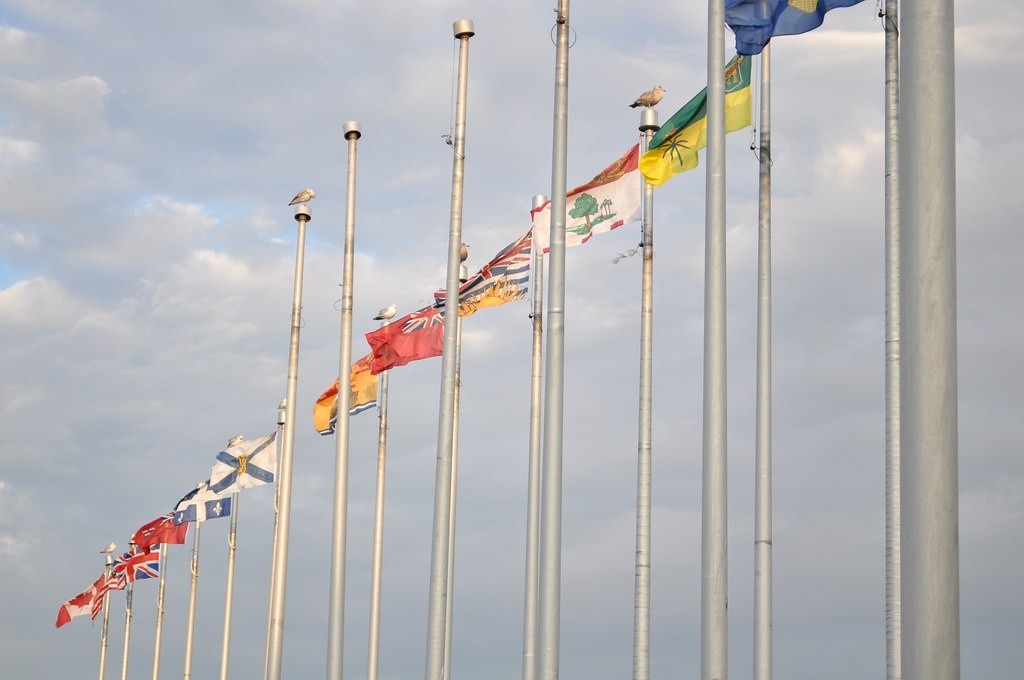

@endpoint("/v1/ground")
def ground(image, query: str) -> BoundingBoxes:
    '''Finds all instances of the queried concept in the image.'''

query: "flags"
[92,571,126,620]
[639,44,752,185]
[56,572,105,628]
[173,478,231,526]
[725,0,867,56]
[314,351,377,435]
[365,303,444,375]
[132,512,189,555]
[433,227,530,321]
[530,144,642,253]
[210,432,276,495]
[114,543,160,589]
